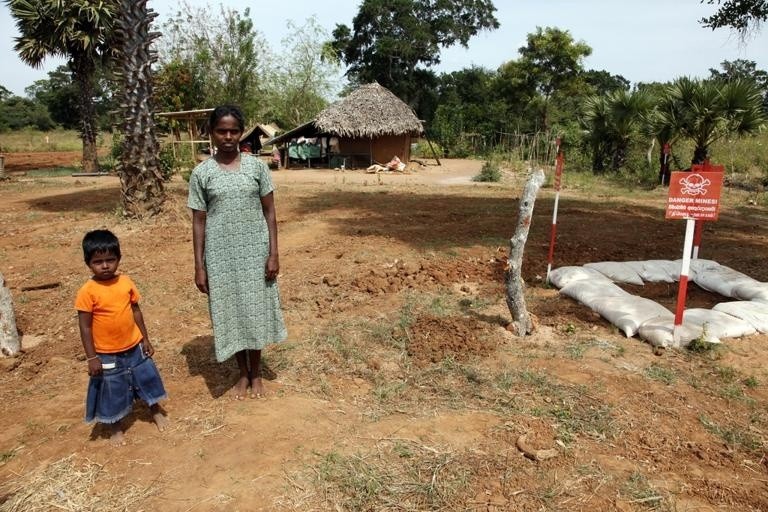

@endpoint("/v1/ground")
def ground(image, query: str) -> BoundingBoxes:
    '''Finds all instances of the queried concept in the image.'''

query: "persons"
[74,229,171,449]
[187,104,281,401]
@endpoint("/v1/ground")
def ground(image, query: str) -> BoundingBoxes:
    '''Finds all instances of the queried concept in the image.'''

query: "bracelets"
[86,356,96,362]
